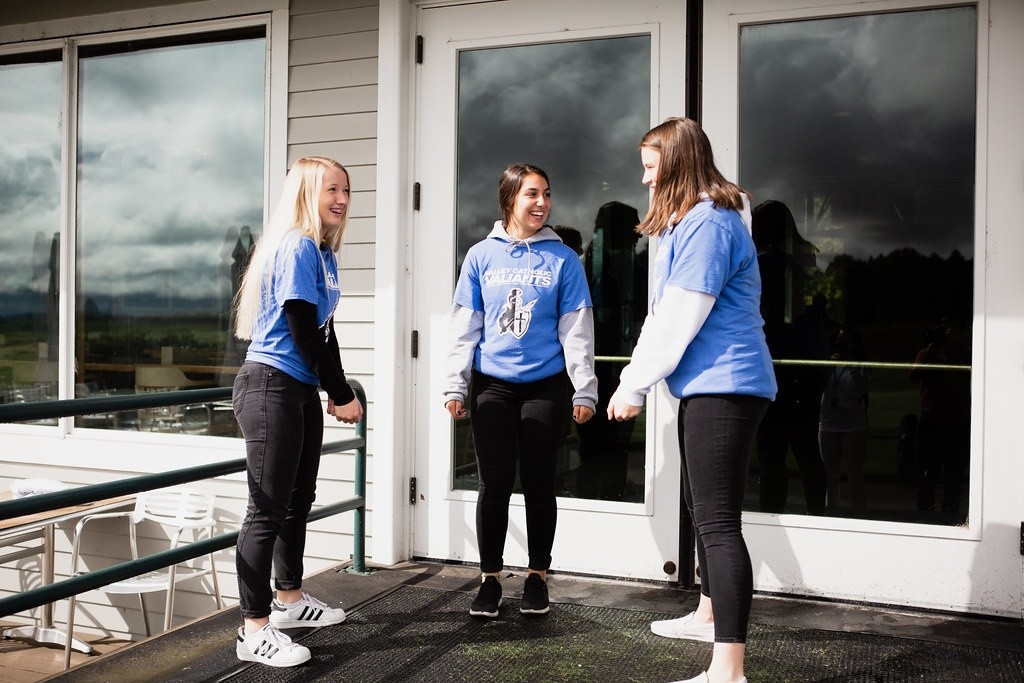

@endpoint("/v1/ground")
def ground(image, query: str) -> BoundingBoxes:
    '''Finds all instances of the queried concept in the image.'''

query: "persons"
[231,156,364,668]
[440,162,598,617]
[607,116,778,683]
[555,202,859,516]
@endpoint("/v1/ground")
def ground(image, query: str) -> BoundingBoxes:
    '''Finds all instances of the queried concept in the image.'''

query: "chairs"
[63,480,220,670]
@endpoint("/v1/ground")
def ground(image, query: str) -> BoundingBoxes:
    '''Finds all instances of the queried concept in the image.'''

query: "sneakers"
[236,622,311,667]
[469,575,503,616]
[519,573,550,614]
[269,592,346,629]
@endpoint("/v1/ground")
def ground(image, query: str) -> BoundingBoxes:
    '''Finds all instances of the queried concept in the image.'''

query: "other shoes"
[650,611,715,642]
[675,671,748,683]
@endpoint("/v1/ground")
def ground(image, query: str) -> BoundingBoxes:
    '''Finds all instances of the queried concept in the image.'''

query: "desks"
[0,492,135,658]
